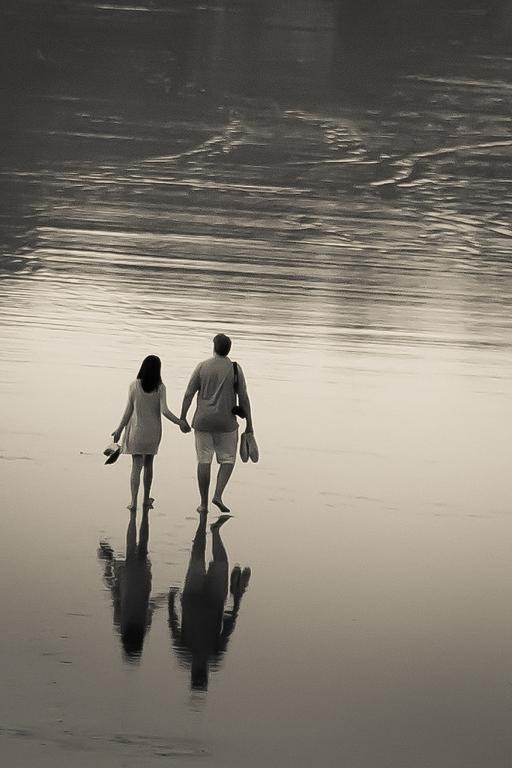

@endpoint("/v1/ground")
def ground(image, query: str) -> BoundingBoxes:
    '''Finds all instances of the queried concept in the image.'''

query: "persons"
[168,513,242,691]
[180,334,253,513]
[104,510,152,657]
[112,355,191,510]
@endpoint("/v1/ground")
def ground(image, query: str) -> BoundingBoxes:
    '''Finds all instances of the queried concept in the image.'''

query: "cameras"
[231,406,246,419]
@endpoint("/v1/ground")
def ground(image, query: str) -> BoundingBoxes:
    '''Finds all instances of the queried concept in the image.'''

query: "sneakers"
[246,432,259,464]
[239,432,248,464]
